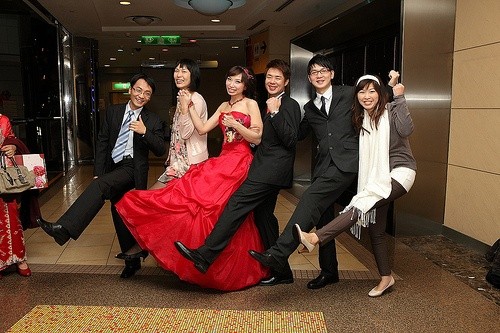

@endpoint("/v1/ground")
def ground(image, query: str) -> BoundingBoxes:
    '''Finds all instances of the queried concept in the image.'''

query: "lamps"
[188,0,233,17]
[132,17,154,25]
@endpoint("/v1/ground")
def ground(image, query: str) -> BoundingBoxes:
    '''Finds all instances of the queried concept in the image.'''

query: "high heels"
[114,249,149,263]
[292,223,316,255]
[367,277,395,297]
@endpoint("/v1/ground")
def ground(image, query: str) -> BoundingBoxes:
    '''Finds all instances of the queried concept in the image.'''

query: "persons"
[174,58,302,284]
[0,114,32,277]
[293,75,416,297]
[114,59,268,290]
[36,75,167,278]
[247,54,400,290]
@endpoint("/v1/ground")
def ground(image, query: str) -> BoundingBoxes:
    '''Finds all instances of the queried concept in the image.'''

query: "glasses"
[308,67,334,76]
[132,87,152,97]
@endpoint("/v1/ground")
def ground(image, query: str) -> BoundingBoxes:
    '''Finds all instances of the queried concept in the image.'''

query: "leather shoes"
[16,262,32,277]
[248,249,272,266]
[119,261,142,279]
[257,274,294,286]
[36,217,71,246]
[174,240,211,274]
[307,267,339,290]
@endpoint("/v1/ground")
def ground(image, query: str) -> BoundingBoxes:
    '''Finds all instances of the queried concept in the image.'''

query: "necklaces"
[229,96,245,108]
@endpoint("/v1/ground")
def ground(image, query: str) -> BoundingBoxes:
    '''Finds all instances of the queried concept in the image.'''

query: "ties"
[320,96,328,117]
[111,110,135,164]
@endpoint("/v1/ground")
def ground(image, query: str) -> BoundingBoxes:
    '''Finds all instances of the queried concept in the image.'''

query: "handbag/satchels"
[0,151,36,194]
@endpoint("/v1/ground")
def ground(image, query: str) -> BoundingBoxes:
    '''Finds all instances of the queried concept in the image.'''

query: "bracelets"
[188,101,194,108]
